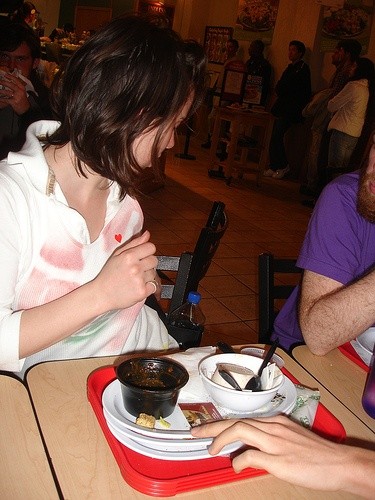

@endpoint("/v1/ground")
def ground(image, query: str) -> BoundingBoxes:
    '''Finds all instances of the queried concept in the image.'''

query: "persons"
[265,41,313,180]
[303,40,374,208]
[0,0,213,156]
[271,129,375,355]
[203,39,242,158]
[0,15,201,378]
[243,39,272,107]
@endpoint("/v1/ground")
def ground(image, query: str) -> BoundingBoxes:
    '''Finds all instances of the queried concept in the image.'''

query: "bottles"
[168,291,206,331]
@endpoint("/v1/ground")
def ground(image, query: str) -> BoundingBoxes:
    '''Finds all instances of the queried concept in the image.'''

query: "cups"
[116,357,189,420]
[361,346,375,419]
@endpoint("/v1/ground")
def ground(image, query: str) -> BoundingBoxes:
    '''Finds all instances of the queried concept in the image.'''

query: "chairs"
[150,201,229,326]
[257,252,305,346]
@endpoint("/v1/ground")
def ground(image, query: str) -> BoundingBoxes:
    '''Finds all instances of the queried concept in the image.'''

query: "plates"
[226,105,248,111]
[321,7,369,37]
[239,15,274,30]
[101,353,297,461]
[240,348,284,369]
[350,340,374,367]
[356,327,375,355]
[250,110,269,113]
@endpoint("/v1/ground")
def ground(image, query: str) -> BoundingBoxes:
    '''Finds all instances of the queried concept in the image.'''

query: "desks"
[0,344,375,500]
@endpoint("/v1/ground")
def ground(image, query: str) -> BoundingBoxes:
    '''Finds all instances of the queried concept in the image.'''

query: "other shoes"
[264,165,291,178]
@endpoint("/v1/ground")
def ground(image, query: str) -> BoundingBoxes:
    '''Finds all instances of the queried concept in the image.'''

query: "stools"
[208,108,273,190]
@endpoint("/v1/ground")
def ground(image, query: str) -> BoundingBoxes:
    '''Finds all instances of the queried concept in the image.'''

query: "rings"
[149,281,159,294]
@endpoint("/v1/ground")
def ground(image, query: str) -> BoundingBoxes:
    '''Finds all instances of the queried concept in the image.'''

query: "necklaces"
[191,414,375,491]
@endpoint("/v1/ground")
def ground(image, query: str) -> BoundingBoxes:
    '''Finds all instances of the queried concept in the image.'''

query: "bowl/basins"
[198,353,284,412]
